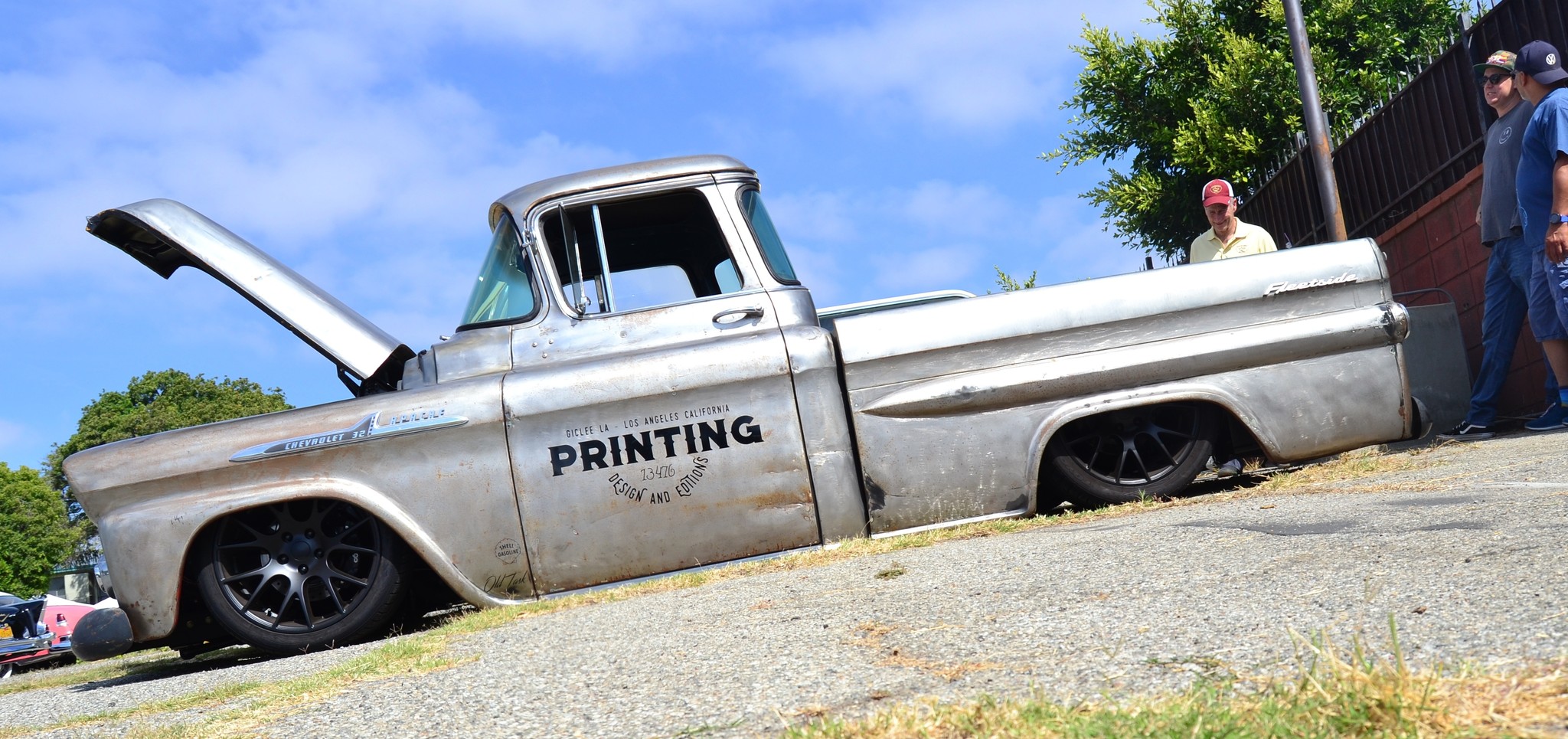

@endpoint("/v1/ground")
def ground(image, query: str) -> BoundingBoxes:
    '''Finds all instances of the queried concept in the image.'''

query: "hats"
[1202,179,1234,208]
[1473,50,1517,74]
[1515,40,1568,85]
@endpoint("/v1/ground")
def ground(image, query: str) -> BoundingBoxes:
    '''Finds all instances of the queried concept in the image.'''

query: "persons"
[1190,179,1278,477]
[1510,40,1568,432]
[1438,50,1533,441]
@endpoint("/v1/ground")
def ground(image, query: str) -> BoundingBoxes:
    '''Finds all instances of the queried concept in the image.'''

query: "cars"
[0,589,119,680]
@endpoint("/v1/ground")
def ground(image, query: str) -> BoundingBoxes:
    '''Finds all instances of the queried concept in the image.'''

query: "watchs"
[1549,212,1568,224]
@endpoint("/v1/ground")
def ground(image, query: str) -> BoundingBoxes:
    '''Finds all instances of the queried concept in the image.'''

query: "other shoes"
[1218,465,1241,478]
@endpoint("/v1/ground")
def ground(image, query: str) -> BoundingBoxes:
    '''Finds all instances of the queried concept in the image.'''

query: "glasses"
[1478,73,1514,86]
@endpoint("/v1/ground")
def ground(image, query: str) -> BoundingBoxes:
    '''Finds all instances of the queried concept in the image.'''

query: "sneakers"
[1524,403,1568,430]
[1436,420,1497,441]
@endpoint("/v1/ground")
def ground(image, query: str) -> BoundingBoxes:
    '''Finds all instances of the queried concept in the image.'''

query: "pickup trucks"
[61,154,1433,663]
